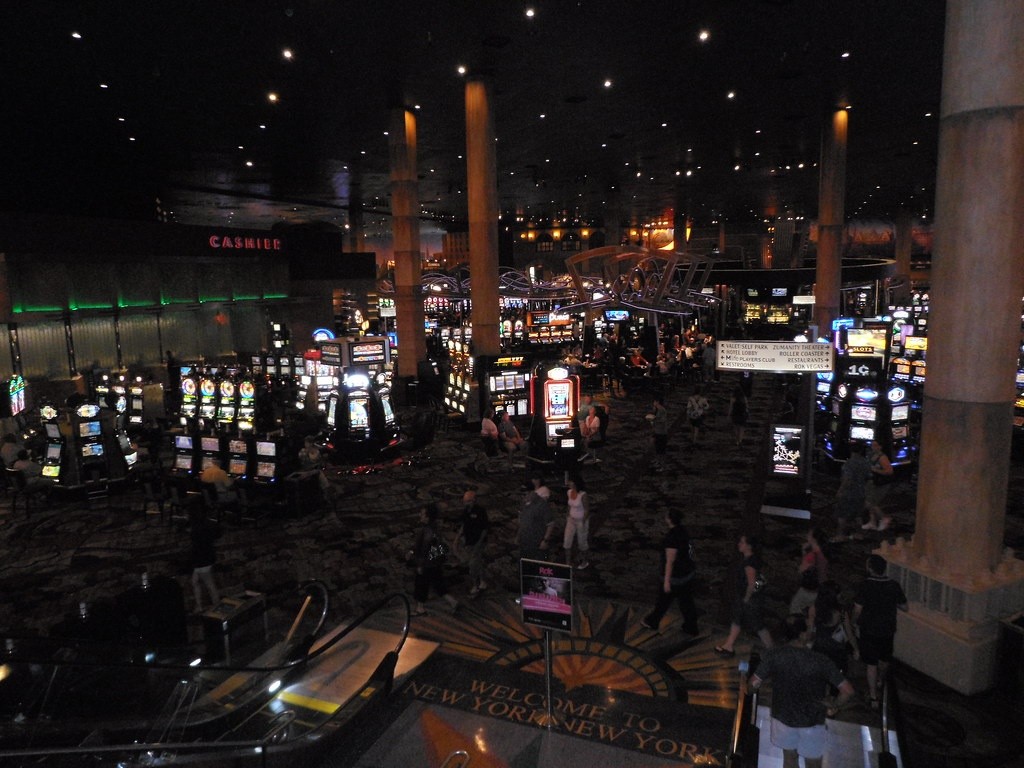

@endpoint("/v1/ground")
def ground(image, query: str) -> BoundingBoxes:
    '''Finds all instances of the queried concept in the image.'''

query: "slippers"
[715,646,735,656]
[410,609,427,617]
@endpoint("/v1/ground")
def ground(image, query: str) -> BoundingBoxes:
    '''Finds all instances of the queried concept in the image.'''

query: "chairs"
[6,468,39,518]
[581,406,608,464]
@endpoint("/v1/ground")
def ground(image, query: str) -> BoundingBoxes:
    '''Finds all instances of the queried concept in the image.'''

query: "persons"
[0,433,48,488]
[406,503,462,615]
[201,454,237,502]
[514,472,590,601]
[644,398,667,471]
[728,386,748,444]
[474,394,609,472]
[716,533,909,768]
[189,497,219,611]
[829,439,892,541]
[561,319,720,392]
[453,490,488,593]
[687,390,708,441]
[639,507,700,635]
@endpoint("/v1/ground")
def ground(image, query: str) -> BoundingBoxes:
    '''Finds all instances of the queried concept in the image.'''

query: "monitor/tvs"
[603,307,632,323]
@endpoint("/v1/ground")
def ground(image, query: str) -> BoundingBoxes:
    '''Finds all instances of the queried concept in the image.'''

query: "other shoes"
[466,589,480,600]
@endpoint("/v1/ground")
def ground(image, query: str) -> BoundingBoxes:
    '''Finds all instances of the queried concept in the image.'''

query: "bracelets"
[542,538,549,543]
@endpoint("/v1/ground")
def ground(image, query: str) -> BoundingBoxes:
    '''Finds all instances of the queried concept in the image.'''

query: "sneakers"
[861,522,877,530]
[877,518,892,531]
[578,561,589,570]
